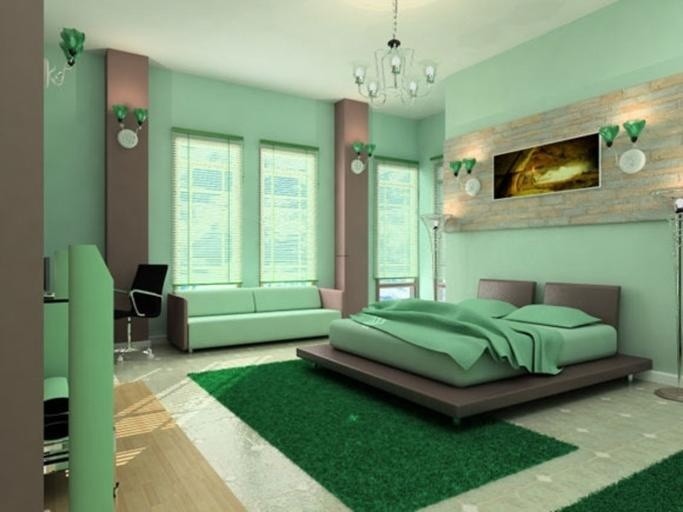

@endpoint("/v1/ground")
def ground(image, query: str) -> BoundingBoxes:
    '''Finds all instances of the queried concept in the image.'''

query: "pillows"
[456,296,517,317]
[499,302,602,329]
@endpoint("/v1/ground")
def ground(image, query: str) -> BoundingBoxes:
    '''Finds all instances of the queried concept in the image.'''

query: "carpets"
[558,447,682,512]
[187,358,579,511]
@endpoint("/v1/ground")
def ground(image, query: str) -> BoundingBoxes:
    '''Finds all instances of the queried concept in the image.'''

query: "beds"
[295,279,652,428]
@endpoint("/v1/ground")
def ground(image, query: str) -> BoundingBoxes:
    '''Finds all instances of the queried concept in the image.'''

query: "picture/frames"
[491,130,600,201]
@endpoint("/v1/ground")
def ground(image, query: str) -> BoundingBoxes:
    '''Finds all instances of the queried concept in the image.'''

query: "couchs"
[166,282,345,355]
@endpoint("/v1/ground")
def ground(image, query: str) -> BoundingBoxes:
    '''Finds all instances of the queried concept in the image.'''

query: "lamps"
[43,27,87,92]
[348,0,440,110]
[447,154,484,196]
[420,212,455,302]
[598,117,648,176]
[348,142,375,175]
[652,196,682,406]
[112,104,148,149]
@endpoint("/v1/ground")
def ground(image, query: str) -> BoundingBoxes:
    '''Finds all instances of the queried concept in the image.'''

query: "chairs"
[113,263,169,363]
